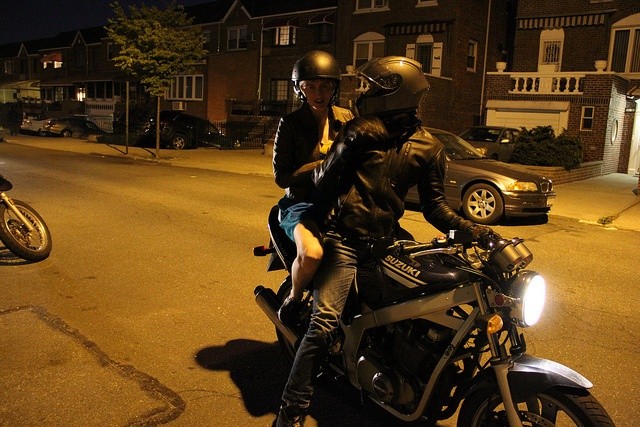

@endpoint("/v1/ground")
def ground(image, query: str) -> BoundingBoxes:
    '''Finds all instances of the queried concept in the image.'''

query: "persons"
[276,56,504,427]
[274,50,352,323]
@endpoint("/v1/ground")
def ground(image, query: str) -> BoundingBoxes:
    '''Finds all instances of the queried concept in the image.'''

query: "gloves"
[466,223,503,250]
[341,115,390,151]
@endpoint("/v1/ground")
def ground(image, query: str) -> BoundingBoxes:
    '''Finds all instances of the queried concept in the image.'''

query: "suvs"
[141,111,222,148]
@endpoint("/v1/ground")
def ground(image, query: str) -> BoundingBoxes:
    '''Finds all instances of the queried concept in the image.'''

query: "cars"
[20,115,55,135]
[458,125,518,157]
[50,118,100,136]
[399,128,555,224]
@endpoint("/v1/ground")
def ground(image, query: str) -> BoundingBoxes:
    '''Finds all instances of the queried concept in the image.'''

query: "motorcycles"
[253,204,610,427]
[0,175,52,262]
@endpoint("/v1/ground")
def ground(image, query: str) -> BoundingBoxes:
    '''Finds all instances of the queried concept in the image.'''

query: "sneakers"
[275,401,310,426]
[278,295,300,323]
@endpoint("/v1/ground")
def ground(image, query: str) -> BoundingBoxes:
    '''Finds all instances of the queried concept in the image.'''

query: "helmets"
[291,49,342,102]
[354,55,431,117]
